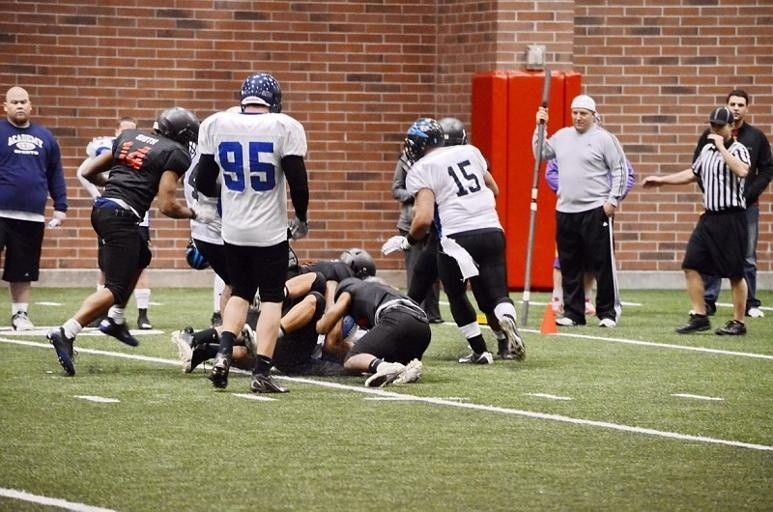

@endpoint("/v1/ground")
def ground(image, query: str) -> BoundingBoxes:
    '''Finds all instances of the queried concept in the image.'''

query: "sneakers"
[583,301,596,316]
[555,318,577,325]
[211,312,222,327]
[10,311,35,331]
[689,308,714,316]
[240,323,259,361]
[98,317,138,347]
[457,350,494,365]
[499,314,527,363]
[170,324,204,374]
[45,326,76,376]
[497,339,511,361]
[392,357,424,384]
[598,317,617,328]
[363,362,407,388]
[209,352,231,389]
[249,372,291,393]
[747,308,764,318]
[549,301,565,317]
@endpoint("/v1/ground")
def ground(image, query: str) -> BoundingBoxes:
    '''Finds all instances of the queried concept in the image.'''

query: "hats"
[704,106,735,127]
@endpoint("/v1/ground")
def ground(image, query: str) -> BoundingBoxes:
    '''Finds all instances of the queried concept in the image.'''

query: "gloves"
[186,242,211,270]
[379,235,413,257]
[189,203,218,225]
[286,216,308,239]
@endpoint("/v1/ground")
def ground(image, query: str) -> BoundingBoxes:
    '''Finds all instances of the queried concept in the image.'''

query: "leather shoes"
[428,318,444,323]
[715,321,746,336]
[676,316,711,334]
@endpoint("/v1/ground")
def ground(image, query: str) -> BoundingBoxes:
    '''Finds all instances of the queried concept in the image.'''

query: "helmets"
[406,118,445,157]
[151,107,200,148]
[339,246,376,278]
[238,73,283,113]
[438,118,466,146]
[333,277,361,302]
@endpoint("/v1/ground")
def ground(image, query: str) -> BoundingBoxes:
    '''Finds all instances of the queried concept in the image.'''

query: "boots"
[136,308,152,329]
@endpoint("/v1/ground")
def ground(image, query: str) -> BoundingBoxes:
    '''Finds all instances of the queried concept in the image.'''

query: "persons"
[0,86,69,332]
[86,117,154,330]
[545,110,636,316]
[46,106,217,376]
[641,107,752,336]
[532,93,629,328]
[689,90,773,319]
